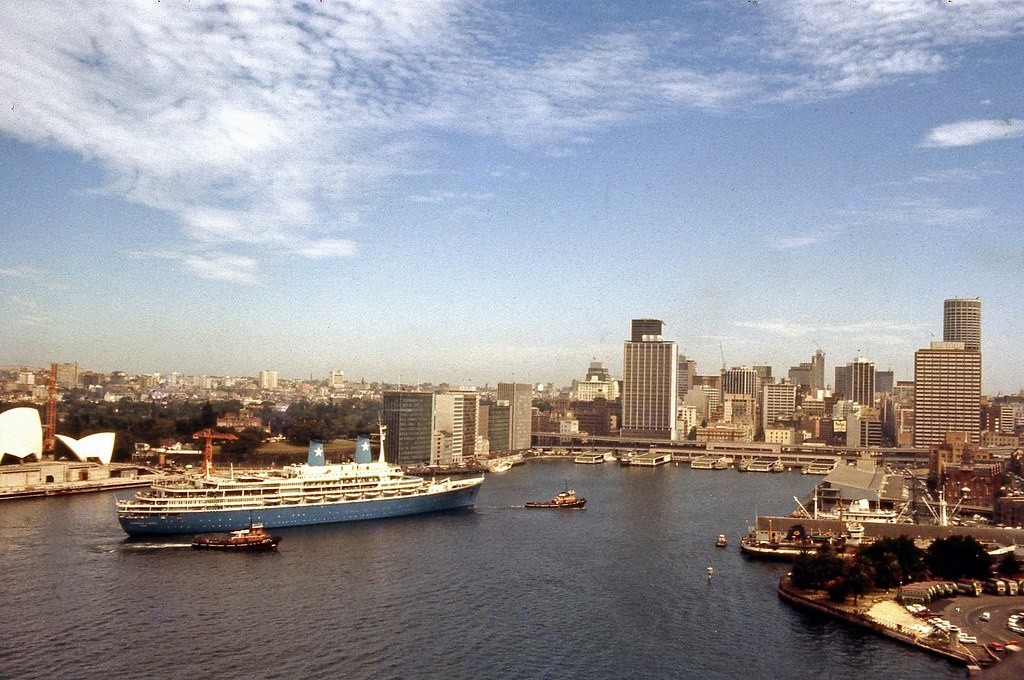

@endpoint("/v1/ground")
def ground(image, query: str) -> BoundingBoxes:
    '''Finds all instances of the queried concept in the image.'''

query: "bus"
[895,572,1024,656]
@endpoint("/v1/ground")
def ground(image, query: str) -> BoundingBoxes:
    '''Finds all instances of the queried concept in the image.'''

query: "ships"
[112,408,487,538]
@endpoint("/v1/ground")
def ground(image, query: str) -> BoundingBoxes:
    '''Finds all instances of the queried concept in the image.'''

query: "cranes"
[191,425,239,473]
[19,363,76,455]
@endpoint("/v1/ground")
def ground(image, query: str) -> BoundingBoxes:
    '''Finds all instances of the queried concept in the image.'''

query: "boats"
[324,494,343,501]
[305,495,323,503]
[523,478,587,508]
[283,497,302,504]
[419,487,429,492]
[191,509,284,551]
[383,489,399,496]
[263,498,282,505]
[345,492,362,500]
[401,487,417,495]
[715,533,728,548]
[364,491,382,498]
